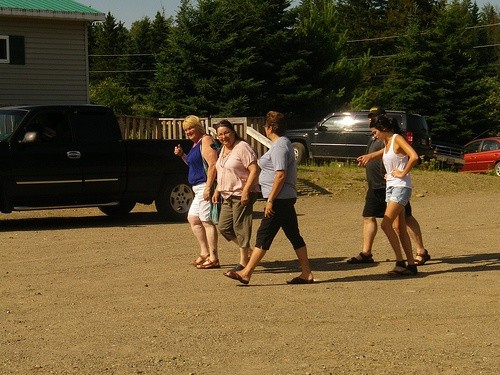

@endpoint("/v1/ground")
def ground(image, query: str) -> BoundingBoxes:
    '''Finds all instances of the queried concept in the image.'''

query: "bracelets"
[181,152,184,157]
[267,199,273,202]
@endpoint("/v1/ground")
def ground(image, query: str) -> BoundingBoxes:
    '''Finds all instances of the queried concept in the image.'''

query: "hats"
[367,106,386,118]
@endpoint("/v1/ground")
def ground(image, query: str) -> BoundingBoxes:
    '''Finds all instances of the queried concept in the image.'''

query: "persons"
[211,120,258,272]
[174,115,221,268]
[222,110,314,285]
[346,107,431,265]
[369,115,419,278]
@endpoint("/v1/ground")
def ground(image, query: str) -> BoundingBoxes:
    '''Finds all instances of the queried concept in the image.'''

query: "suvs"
[284,110,435,166]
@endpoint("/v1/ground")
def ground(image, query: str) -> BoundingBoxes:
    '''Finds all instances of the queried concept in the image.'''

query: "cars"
[453,137,500,177]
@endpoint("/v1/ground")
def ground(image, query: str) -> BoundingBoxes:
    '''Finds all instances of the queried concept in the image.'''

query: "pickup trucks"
[0,102,220,221]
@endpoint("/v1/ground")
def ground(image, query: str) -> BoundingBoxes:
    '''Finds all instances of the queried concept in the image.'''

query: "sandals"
[190,254,210,264]
[347,253,374,264]
[196,259,220,269]
[413,249,430,265]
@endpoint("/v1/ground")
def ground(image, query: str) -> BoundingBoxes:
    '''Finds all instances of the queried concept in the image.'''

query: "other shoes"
[399,265,418,277]
[235,263,245,271]
[388,261,407,276]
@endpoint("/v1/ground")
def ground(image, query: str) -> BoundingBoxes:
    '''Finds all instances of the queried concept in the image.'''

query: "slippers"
[223,270,249,284]
[287,277,314,284]
